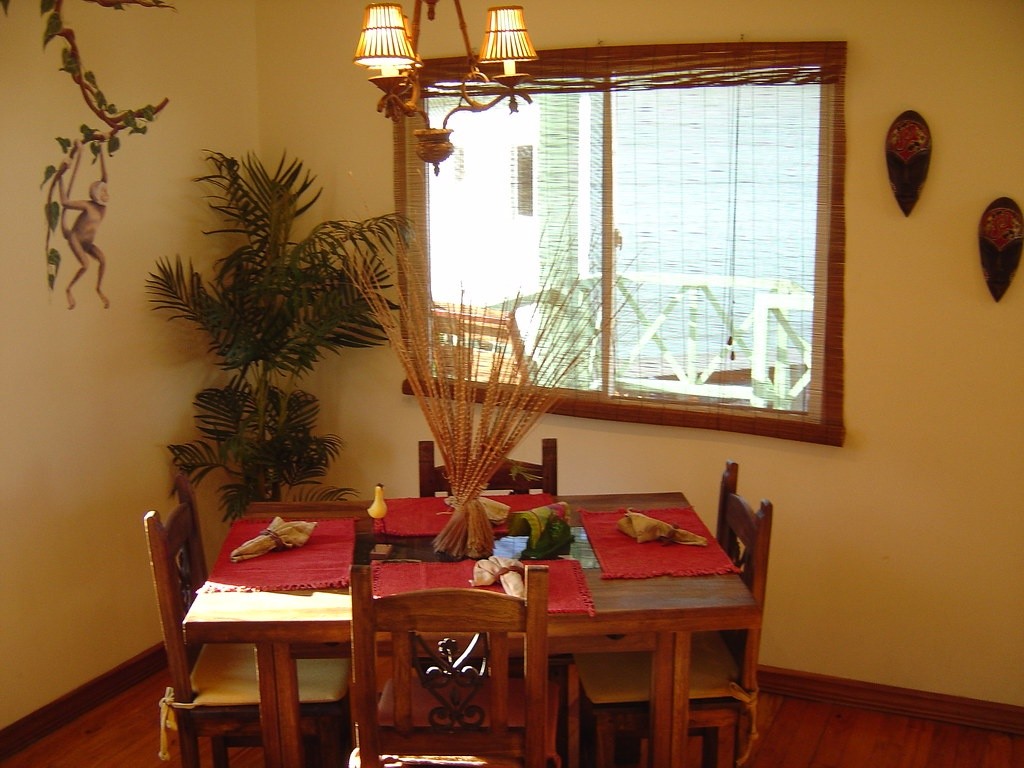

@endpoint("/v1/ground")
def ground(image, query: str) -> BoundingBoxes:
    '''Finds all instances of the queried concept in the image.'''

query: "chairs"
[417,439,559,502]
[578,459,775,767]
[345,563,561,767]
[144,492,347,768]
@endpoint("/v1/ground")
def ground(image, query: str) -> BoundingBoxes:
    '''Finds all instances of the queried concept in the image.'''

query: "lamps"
[353,0,539,176]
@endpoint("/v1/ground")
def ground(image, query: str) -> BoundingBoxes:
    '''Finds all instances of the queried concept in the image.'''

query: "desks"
[183,493,761,768]
